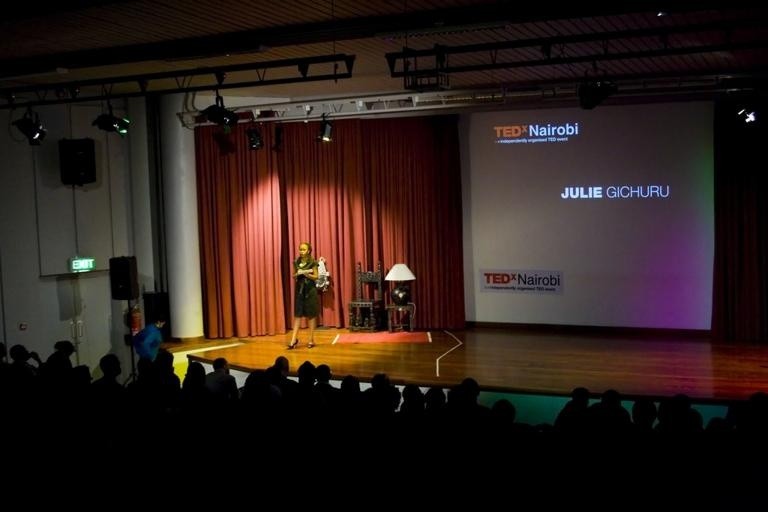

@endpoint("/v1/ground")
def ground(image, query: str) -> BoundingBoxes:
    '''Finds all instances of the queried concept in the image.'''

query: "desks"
[384,304,416,332]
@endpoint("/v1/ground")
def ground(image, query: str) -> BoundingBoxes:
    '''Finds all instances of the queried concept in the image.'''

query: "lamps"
[92,98,131,138]
[12,105,47,146]
[247,122,264,152]
[201,89,238,127]
[383,264,417,304]
[317,116,333,142]
[579,82,618,110]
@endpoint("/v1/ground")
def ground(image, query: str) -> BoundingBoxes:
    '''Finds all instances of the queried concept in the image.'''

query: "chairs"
[348,262,387,332]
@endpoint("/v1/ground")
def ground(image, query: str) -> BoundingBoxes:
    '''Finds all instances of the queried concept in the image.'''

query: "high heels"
[308,342,314,348]
[288,338,298,348]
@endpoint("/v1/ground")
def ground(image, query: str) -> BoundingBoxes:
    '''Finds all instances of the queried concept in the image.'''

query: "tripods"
[123,300,138,387]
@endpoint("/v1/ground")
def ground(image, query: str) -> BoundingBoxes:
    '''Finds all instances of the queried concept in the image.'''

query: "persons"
[287,243,322,348]
[134,318,166,364]
[0,341,768,512]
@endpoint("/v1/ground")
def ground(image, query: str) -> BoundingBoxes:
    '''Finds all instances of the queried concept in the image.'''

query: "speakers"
[109,256,139,300]
[58,138,96,185]
